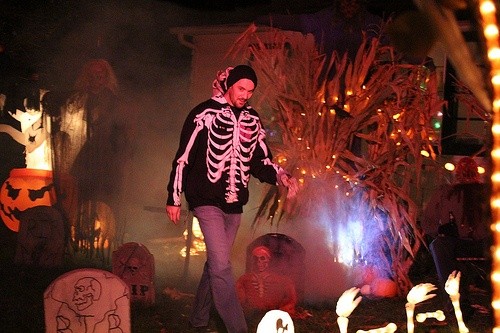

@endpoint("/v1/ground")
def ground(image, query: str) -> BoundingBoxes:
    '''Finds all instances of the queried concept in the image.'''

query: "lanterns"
[70,199,117,247]
[0,167,80,236]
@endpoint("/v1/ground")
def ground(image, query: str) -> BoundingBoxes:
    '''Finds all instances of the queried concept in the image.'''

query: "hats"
[226,64,258,89]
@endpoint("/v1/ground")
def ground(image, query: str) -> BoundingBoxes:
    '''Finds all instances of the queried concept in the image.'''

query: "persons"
[165,65,300,333]
